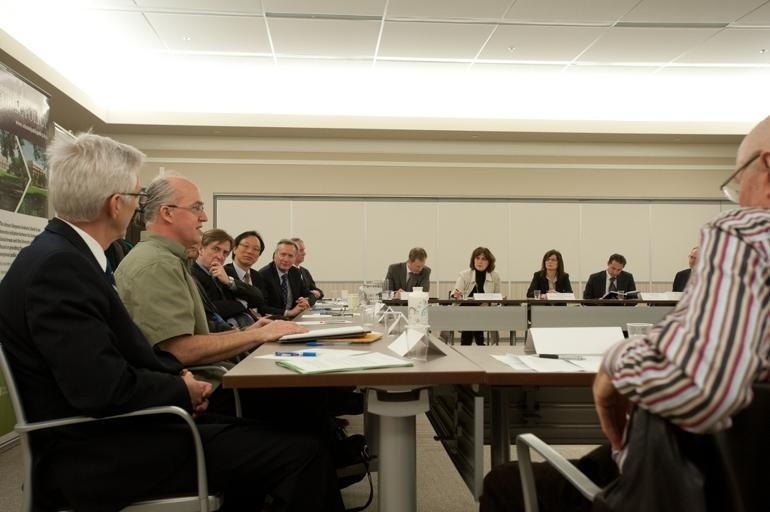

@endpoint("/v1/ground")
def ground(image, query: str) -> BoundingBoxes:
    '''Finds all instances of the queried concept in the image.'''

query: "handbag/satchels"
[331,432,370,491]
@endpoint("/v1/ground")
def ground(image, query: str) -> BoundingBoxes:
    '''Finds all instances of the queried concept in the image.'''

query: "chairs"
[3,345,242,509]
[376,276,680,348]
[515,380,768,508]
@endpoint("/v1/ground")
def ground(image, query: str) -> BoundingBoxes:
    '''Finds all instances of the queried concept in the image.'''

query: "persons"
[188,229,325,332]
[113,175,309,425]
[381,247,431,300]
[0,135,344,512]
[583,253,638,300]
[673,246,698,292]
[448,247,501,346]
[526,249,573,325]
[478,116,770,512]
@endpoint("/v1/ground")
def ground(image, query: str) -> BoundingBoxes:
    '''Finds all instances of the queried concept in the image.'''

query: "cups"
[618,290,624,301]
[383,312,400,337]
[625,322,653,337]
[361,301,376,330]
[382,280,394,301]
[534,290,540,300]
[405,324,428,362]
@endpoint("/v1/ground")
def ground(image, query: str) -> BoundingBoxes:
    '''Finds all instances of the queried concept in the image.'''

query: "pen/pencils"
[309,307,330,310]
[305,341,352,346]
[274,351,318,357]
[456,289,464,298]
[535,354,583,360]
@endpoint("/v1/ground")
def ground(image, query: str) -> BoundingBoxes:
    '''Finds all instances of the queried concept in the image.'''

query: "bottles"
[408,286,429,324]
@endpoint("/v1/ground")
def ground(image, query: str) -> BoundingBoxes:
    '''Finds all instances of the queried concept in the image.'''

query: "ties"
[609,277,617,291]
[281,273,288,308]
[105,258,119,298]
[244,273,250,285]
[407,272,417,291]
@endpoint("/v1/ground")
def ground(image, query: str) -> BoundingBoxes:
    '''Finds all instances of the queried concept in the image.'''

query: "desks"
[223,297,487,510]
[451,343,620,471]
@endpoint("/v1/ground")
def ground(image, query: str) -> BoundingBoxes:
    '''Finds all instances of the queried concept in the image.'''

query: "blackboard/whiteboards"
[212,193,744,285]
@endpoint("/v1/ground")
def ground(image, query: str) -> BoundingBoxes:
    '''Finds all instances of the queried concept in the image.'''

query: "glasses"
[159,202,204,215]
[111,190,150,208]
[719,154,760,204]
[238,242,262,256]
